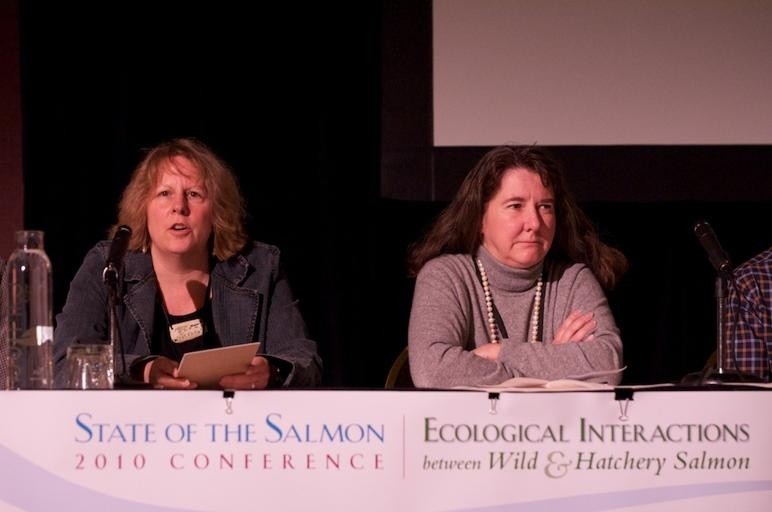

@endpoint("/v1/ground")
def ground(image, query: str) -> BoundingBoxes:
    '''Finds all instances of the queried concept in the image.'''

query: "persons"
[407,144,630,385]
[722,248,771,383]
[44,138,324,391]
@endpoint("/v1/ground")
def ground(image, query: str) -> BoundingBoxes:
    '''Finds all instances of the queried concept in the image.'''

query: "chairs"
[385,346,417,390]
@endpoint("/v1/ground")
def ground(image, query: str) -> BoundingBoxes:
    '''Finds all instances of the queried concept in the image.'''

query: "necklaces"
[476,258,545,344]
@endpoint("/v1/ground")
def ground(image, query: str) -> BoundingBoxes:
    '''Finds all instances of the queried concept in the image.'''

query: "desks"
[0,382,772,512]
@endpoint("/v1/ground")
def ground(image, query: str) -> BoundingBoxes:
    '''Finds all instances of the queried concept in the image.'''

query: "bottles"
[6,228,57,391]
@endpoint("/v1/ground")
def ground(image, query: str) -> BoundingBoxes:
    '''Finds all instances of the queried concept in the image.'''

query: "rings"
[252,382,255,389]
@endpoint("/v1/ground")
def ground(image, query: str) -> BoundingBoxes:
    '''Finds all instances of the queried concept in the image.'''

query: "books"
[175,341,261,390]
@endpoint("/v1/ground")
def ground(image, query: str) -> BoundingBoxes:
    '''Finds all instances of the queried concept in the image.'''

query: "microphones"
[103,224,132,283]
[692,217,732,274]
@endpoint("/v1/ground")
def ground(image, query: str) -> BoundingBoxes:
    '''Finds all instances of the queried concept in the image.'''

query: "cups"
[64,342,115,391]
